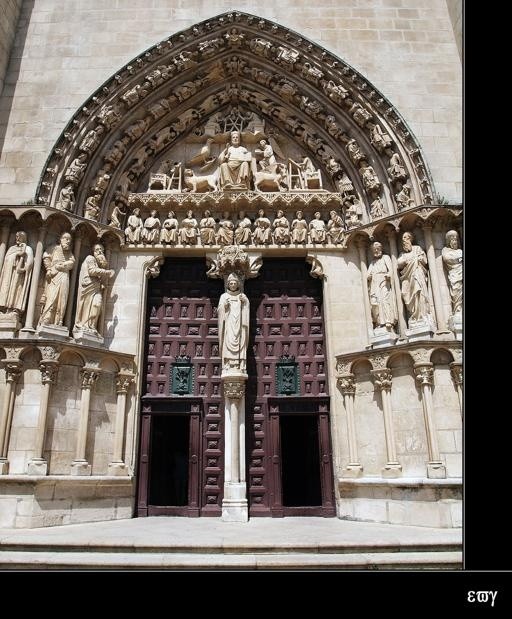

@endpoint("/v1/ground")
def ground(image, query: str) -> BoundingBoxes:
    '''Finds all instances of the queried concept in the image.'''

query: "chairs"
[147,167,166,195]
[205,125,215,136]
[304,169,323,191]
[252,119,265,135]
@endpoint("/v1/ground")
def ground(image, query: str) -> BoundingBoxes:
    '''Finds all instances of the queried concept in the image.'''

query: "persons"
[217,272,251,371]
[55,28,415,246]
[441,230,463,315]
[37,232,75,326]
[0,231,34,316]
[367,241,398,327]
[397,232,435,321]
[73,243,115,335]
